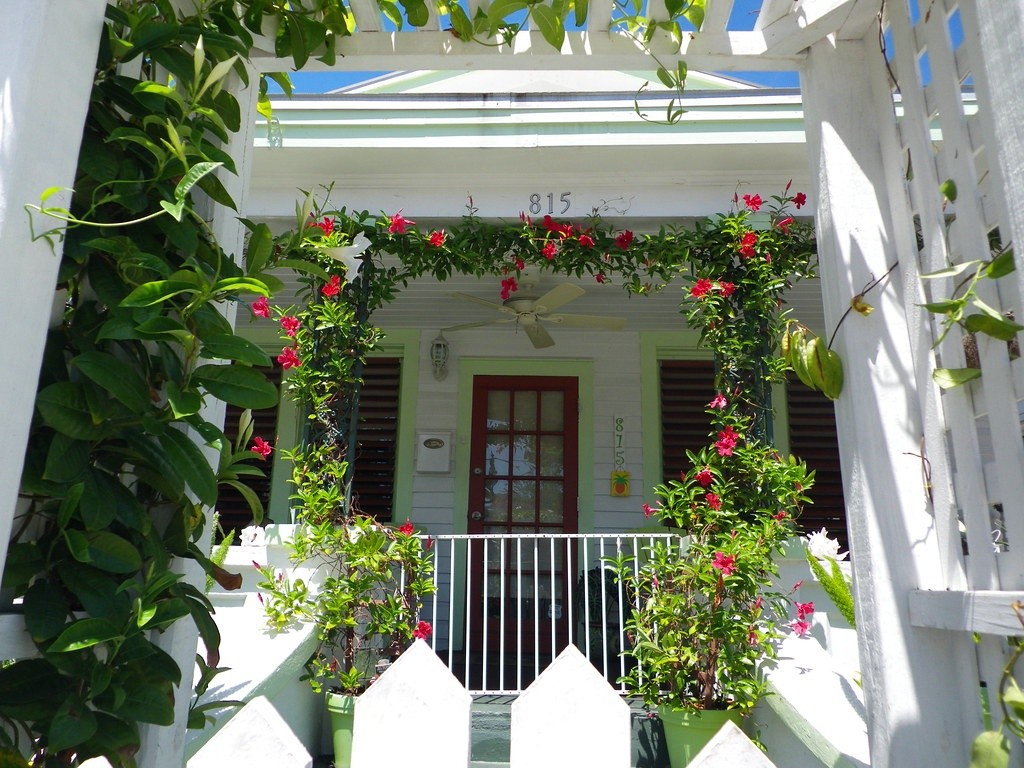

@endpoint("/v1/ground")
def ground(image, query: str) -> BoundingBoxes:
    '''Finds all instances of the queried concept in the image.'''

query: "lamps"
[432,331,450,383]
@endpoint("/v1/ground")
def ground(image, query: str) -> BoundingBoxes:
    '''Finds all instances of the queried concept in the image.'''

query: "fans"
[438,274,625,355]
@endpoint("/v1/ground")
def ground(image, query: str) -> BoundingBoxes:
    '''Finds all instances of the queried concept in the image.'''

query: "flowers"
[247,176,826,702]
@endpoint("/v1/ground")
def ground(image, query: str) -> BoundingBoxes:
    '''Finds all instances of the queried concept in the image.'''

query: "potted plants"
[657,693,746,768]
[324,686,354,768]
